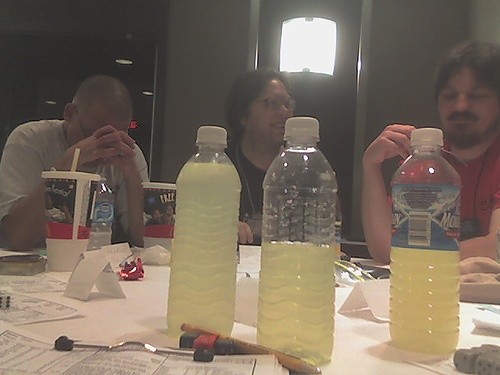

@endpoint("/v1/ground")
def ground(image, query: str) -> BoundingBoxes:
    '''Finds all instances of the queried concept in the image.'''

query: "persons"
[146,204,176,225]
[361,36,500,264]
[0,74,151,251]
[220,67,296,245]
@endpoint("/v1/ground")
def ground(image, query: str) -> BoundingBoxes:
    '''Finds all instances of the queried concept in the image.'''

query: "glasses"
[254,98,296,112]
[75,105,95,138]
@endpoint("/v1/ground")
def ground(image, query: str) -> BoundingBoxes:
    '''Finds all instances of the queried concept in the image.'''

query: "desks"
[0,246,500,375]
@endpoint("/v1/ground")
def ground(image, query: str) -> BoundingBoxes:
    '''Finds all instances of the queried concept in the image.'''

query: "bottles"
[89,178,115,249]
[257,117,337,367]
[389,128,461,353]
[167,126,243,339]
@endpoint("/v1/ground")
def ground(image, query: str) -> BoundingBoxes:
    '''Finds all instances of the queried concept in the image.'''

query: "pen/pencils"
[181,322,324,375]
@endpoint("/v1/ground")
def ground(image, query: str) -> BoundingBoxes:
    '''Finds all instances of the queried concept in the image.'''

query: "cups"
[141,182,179,256]
[42,171,101,272]
